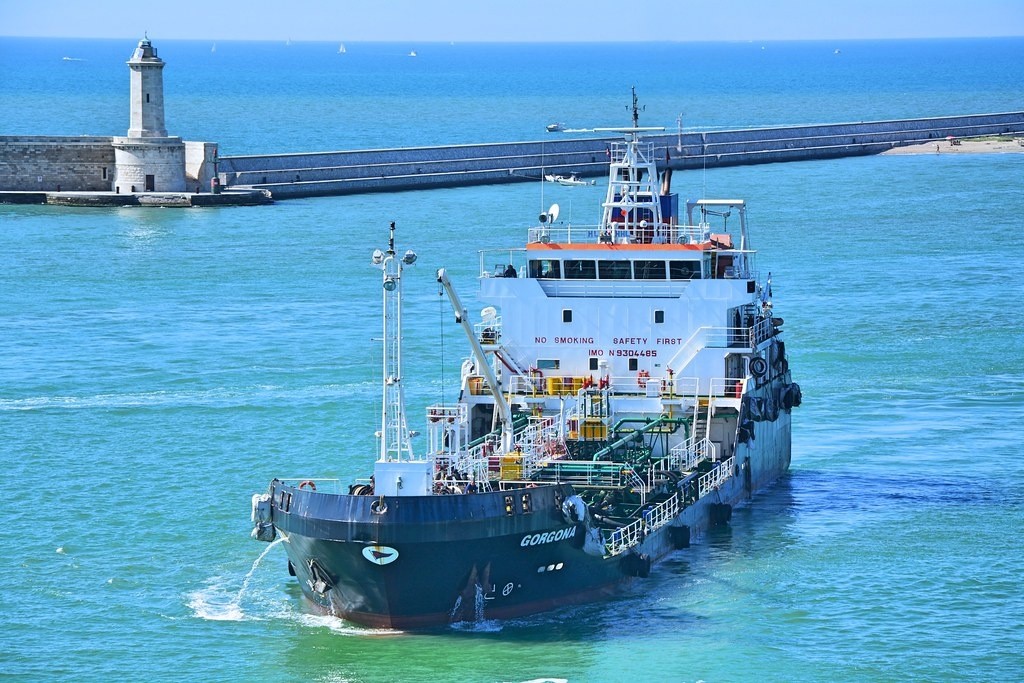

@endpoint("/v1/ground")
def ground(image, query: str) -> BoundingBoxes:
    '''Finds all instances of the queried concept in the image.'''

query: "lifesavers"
[681,523,694,550]
[638,554,652,580]
[298,479,318,493]
[717,502,734,528]
[635,370,651,390]
[750,356,768,378]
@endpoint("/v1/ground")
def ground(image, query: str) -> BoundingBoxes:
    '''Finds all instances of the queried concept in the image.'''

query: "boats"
[250,86,801,632]
[545,175,564,182]
[558,171,591,187]
[545,123,563,131]
